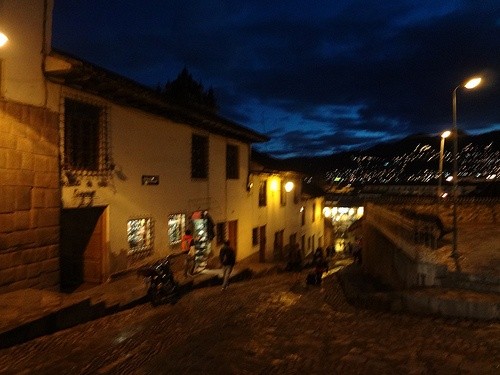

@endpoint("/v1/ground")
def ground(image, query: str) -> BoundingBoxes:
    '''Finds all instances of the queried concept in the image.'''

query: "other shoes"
[190,272,193,275]
[220,286,229,291]
[184,276,187,279]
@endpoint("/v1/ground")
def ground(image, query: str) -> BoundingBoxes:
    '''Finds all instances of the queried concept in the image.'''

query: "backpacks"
[224,247,231,264]
[182,238,188,251]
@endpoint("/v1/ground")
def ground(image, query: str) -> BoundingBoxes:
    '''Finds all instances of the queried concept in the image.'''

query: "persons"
[284,239,354,291]
[220,240,235,291]
[181,230,196,279]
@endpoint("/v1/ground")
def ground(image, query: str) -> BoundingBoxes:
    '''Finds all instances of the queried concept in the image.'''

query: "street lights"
[452,76,483,257]
[437,130,454,199]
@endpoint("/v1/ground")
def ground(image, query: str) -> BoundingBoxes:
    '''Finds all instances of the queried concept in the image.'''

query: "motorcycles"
[138,252,181,306]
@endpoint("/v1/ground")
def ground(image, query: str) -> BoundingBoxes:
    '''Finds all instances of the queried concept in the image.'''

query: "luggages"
[306,271,315,285]
